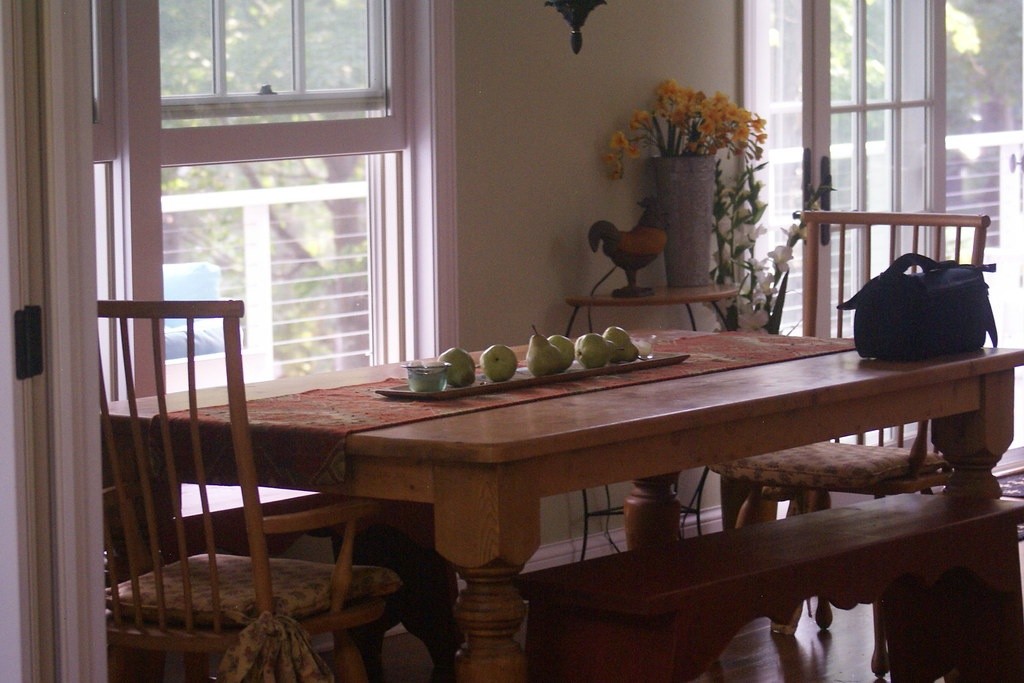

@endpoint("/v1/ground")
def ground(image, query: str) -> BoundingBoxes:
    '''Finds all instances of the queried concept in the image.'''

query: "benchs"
[485,492,1024,683]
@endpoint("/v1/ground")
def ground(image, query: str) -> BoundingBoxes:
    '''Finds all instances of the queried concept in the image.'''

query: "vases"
[650,154,716,287]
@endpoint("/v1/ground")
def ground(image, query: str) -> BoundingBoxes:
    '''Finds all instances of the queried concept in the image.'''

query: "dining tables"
[99,330,1024,682]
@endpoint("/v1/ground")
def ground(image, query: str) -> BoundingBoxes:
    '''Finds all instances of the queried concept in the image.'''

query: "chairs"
[98,301,405,683]
[709,210,1006,679]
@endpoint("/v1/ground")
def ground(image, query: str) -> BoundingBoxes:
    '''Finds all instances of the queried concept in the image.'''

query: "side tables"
[560,283,736,561]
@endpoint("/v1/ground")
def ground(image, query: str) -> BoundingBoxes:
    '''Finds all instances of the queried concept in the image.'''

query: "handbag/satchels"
[836,253,998,363]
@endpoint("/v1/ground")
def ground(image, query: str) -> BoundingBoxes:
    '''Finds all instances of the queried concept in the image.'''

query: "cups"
[401,362,452,392]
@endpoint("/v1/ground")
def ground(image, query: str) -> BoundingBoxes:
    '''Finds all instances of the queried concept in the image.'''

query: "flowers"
[602,79,768,183]
[705,157,836,332]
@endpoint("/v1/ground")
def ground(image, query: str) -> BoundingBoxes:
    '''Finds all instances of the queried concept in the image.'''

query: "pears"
[526,325,563,377]
[479,344,517,381]
[438,348,475,387]
[574,326,644,369]
[546,335,575,372]
